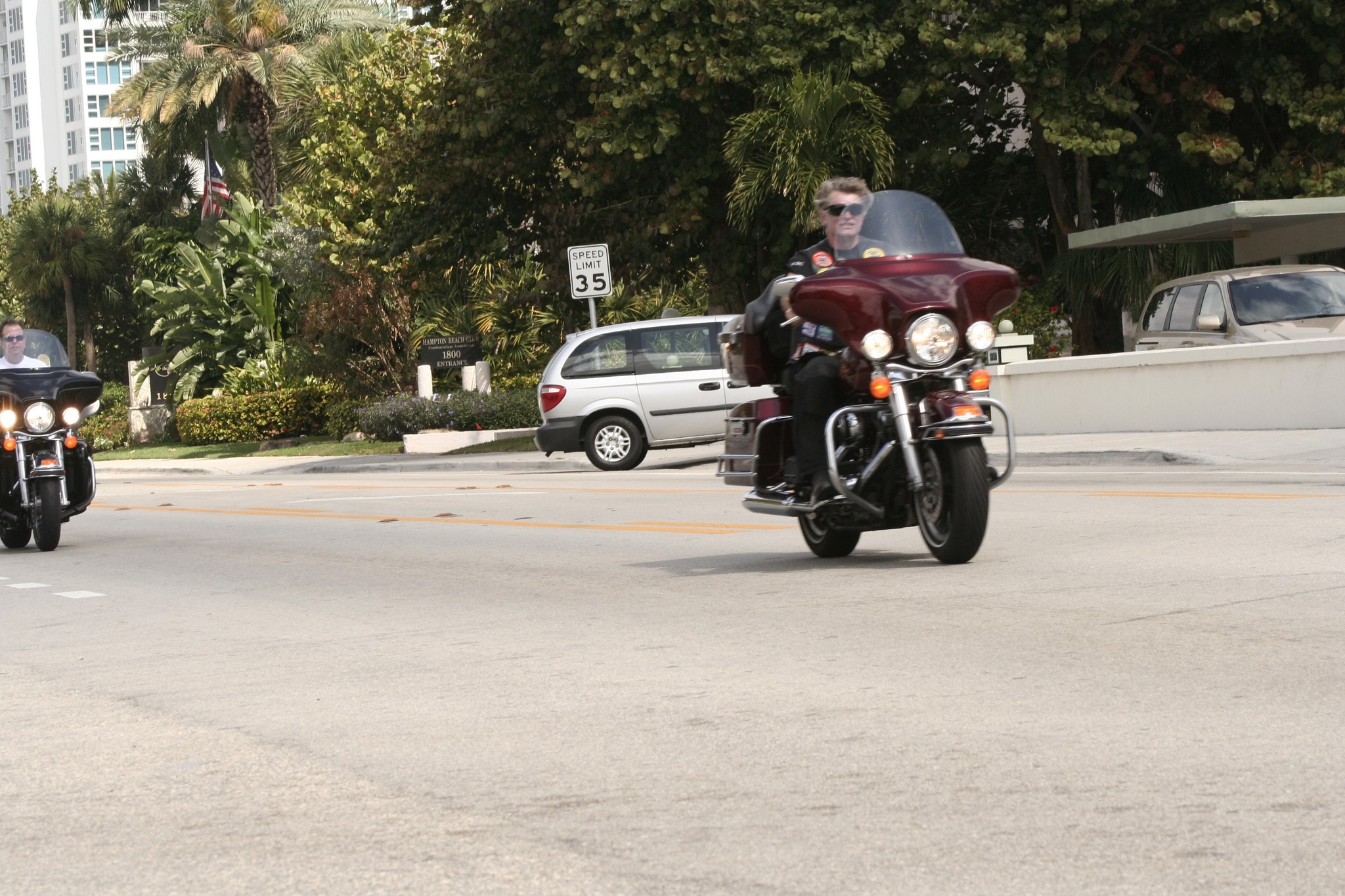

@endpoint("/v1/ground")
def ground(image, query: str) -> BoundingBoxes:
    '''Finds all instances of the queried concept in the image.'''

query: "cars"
[1136,264,1345,358]
[538,316,769,475]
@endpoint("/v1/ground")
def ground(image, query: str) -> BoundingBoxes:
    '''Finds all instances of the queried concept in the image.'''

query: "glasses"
[824,203,864,216]
[3,335,24,342]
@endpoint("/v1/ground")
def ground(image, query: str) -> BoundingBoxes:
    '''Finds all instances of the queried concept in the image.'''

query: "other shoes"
[809,478,831,503]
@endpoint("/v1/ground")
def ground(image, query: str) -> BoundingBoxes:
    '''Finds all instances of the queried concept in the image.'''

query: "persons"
[0,318,49,370]
[780,178,914,502]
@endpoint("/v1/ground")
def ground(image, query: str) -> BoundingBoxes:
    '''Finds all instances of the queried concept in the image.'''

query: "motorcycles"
[0,323,101,556]
[711,189,1032,565]
